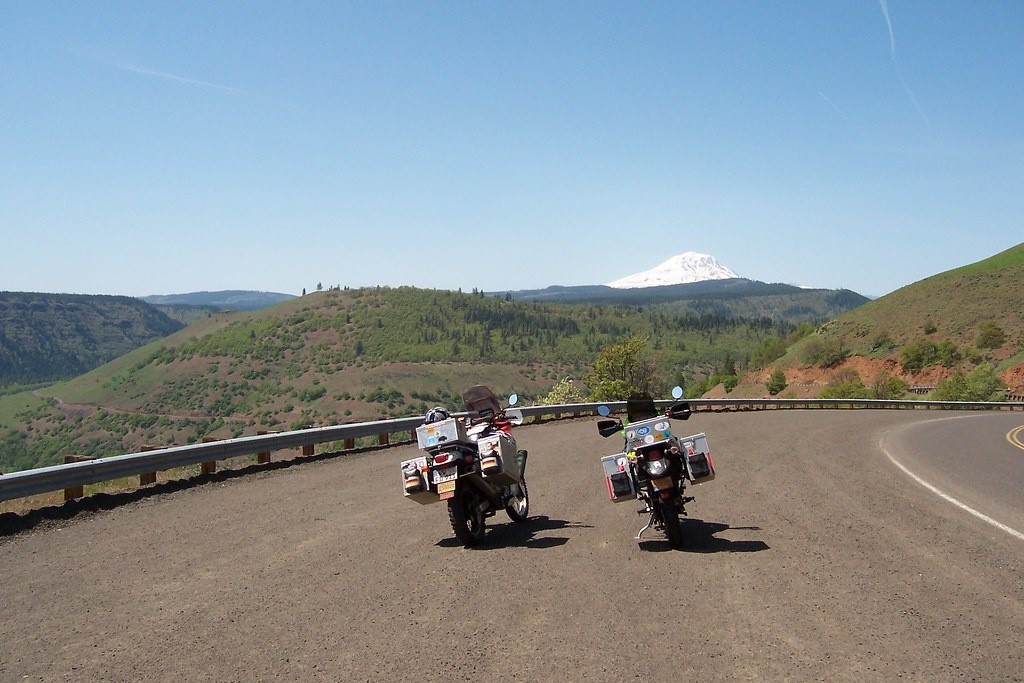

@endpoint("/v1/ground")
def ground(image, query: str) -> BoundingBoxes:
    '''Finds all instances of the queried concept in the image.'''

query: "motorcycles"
[597,385,717,544]
[401,383,529,546]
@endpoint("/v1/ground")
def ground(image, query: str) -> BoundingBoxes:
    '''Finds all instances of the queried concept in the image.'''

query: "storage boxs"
[600,451,636,502]
[680,431,715,485]
[416,417,469,451]
[401,456,442,505]
[623,415,673,451]
[477,435,519,488]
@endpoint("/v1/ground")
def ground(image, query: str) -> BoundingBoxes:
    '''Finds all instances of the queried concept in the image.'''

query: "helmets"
[426,407,450,424]
